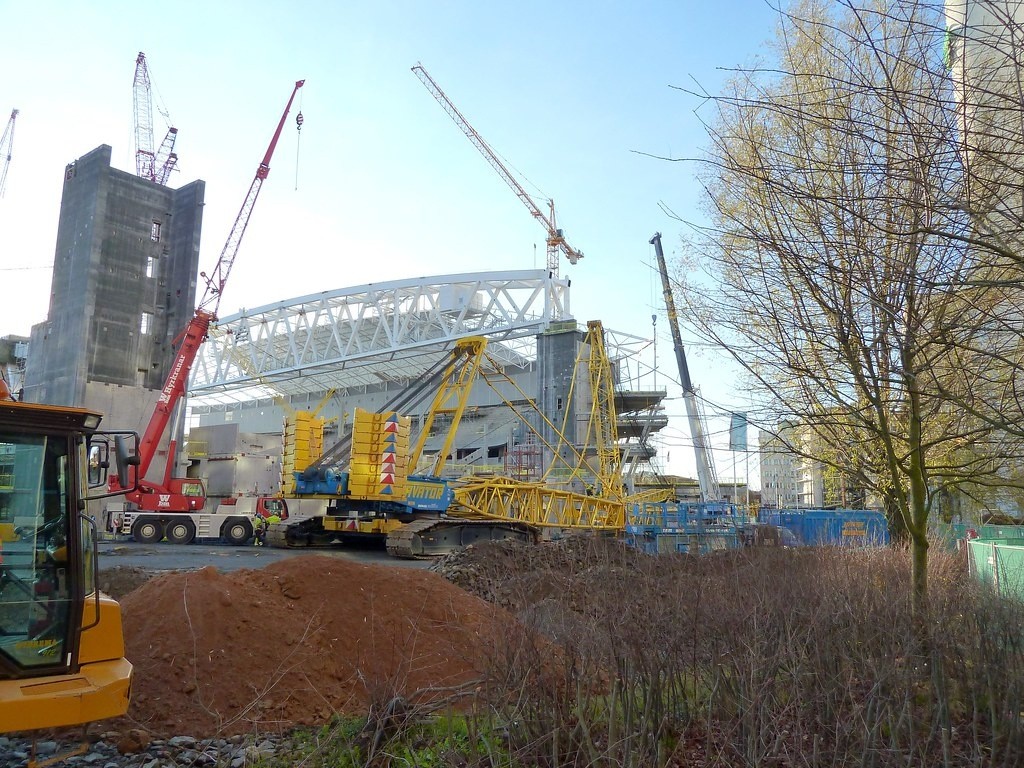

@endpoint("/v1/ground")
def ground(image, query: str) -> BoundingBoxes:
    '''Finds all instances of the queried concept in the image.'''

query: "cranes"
[131,51,181,186]
[410,61,584,321]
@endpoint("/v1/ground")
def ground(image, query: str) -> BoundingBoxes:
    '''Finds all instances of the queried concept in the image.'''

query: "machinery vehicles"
[0,368,132,768]
[261,228,886,572]
[105,78,311,541]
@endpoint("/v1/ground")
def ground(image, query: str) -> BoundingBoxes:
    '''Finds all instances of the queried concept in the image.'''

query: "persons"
[90,460,98,471]
[265,511,281,525]
[586,483,595,496]
[254,514,263,546]
